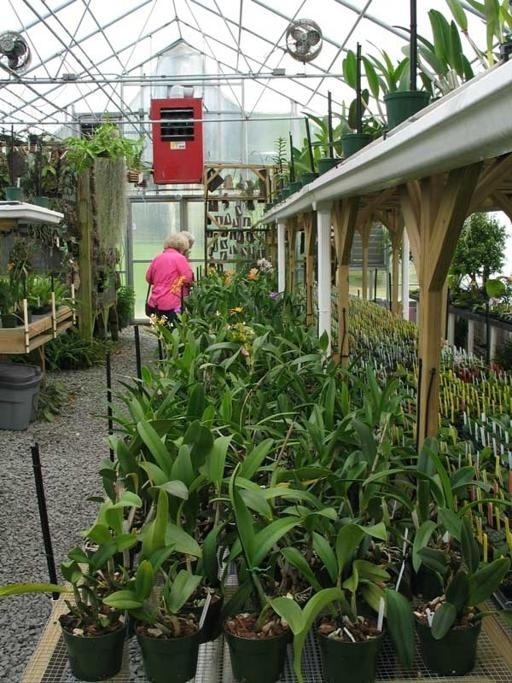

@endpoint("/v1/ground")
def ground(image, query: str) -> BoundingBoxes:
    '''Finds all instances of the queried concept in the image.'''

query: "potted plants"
[262,0,512,212]
[2,258,512,683]
[1,110,135,373]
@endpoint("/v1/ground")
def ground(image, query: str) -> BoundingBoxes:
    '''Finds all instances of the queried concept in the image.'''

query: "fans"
[0,29,32,72]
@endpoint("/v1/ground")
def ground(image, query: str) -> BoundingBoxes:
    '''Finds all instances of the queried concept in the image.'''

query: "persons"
[180,231,194,314]
[143,230,194,359]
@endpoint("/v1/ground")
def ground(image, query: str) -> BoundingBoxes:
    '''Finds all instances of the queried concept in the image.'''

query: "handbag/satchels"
[145,303,159,316]
